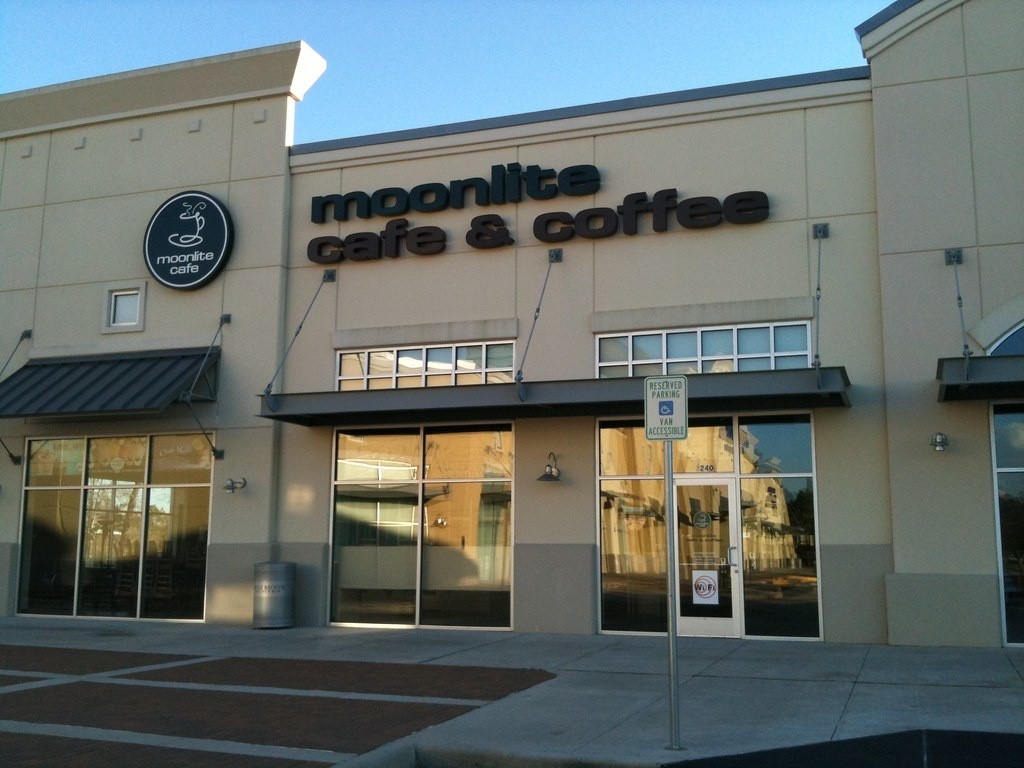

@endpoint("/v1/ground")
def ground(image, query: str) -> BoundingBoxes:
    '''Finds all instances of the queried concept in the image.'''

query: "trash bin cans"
[253,561,297,630]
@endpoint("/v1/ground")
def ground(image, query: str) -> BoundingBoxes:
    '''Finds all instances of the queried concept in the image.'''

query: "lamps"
[223,478,249,494]
[929,432,950,451]
[429,513,448,529]
[539,452,561,482]
